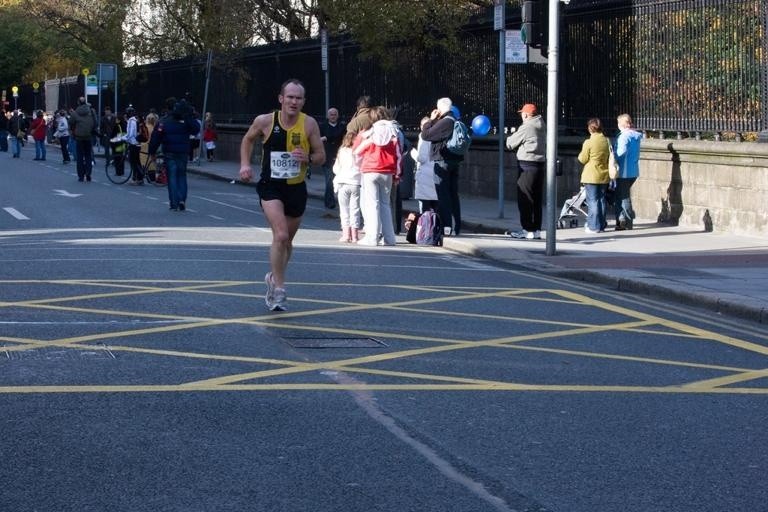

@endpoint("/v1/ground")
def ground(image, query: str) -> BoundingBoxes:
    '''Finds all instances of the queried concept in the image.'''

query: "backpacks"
[135,121,149,143]
[446,120,472,156]
[415,208,442,247]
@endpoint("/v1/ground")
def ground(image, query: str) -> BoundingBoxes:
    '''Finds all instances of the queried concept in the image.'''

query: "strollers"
[556,184,606,231]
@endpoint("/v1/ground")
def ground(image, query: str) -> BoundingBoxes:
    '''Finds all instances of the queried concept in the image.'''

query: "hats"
[517,103,538,116]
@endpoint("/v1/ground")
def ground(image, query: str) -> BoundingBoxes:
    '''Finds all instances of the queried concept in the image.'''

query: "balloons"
[471,114,491,136]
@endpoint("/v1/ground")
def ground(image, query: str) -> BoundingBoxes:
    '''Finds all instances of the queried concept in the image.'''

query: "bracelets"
[308,153,312,166]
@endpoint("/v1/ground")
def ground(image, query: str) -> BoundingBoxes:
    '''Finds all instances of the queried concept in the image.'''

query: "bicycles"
[105,137,168,187]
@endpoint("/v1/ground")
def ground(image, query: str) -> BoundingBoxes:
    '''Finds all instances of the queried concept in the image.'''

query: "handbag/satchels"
[30,128,36,135]
[115,131,129,153]
[607,151,621,180]
[17,129,25,139]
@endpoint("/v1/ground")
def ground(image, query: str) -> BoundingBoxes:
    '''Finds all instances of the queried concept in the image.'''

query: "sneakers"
[531,230,541,239]
[178,201,186,211]
[380,235,398,247]
[357,234,378,247]
[168,203,180,212]
[269,288,289,312]
[265,271,275,306]
[510,228,534,240]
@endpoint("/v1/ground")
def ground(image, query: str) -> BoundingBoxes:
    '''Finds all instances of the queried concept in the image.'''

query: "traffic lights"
[520,0,540,46]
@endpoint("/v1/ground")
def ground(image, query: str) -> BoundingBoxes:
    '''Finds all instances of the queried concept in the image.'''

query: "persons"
[506,104,547,239]
[577,117,610,233]
[0,97,160,185]
[149,98,200,212]
[180,90,218,162]
[239,79,327,312]
[319,96,465,247]
[614,114,643,231]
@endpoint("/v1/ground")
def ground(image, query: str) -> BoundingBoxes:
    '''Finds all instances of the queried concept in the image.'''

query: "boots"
[339,227,351,243]
[350,228,359,243]
